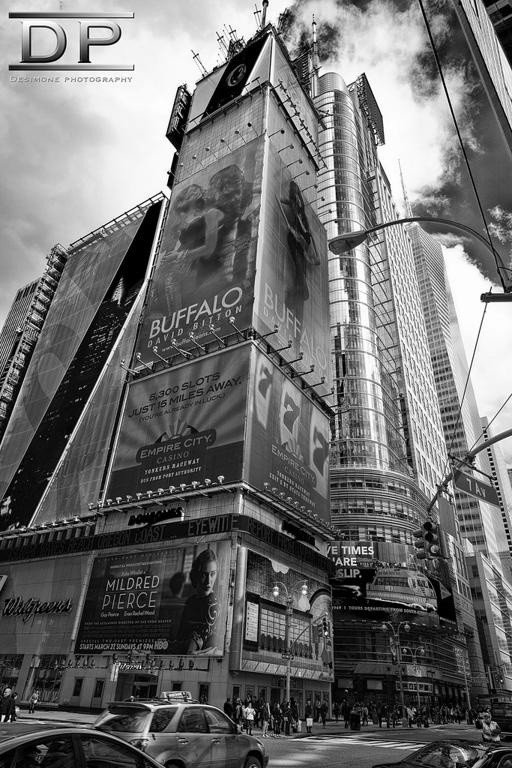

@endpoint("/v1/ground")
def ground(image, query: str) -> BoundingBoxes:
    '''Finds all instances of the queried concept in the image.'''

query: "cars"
[372,738,512,768]
[0,692,269,768]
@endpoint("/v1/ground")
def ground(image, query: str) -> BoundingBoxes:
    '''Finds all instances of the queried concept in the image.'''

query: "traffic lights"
[413,521,442,560]
[317,617,330,639]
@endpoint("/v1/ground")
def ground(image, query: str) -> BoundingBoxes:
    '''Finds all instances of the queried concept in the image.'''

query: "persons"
[223,689,489,739]
[28,690,40,715]
[481,713,502,743]
[1,687,15,722]
[175,549,220,656]
[167,184,231,284]
[276,181,313,328]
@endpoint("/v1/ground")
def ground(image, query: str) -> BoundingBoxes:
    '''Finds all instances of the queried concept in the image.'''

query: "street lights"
[381,621,425,718]
[488,665,504,695]
[271,579,308,708]
[328,217,512,293]
